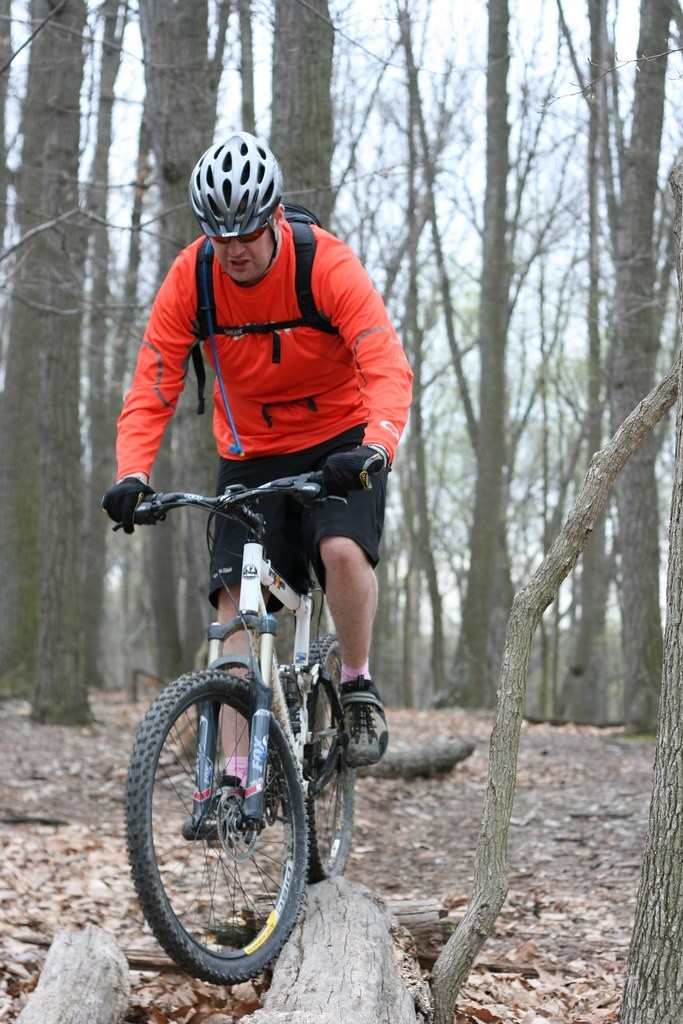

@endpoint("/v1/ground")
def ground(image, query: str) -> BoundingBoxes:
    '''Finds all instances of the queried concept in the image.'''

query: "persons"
[102,131,414,840]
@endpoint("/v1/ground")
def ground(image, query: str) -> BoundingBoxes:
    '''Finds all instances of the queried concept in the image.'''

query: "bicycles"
[112,468,357,988]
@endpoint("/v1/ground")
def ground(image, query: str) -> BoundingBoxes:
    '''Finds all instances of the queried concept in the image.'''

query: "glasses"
[212,213,273,244]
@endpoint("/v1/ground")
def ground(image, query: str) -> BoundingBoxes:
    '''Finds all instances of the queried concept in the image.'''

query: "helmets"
[189,132,283,236]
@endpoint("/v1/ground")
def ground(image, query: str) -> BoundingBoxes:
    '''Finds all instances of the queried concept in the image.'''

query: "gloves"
[101,477,166,534]
[322,444,389,501]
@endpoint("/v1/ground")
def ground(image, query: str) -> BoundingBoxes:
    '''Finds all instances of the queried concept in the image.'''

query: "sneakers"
[341,674,388,768]
[182,775,244,840]
